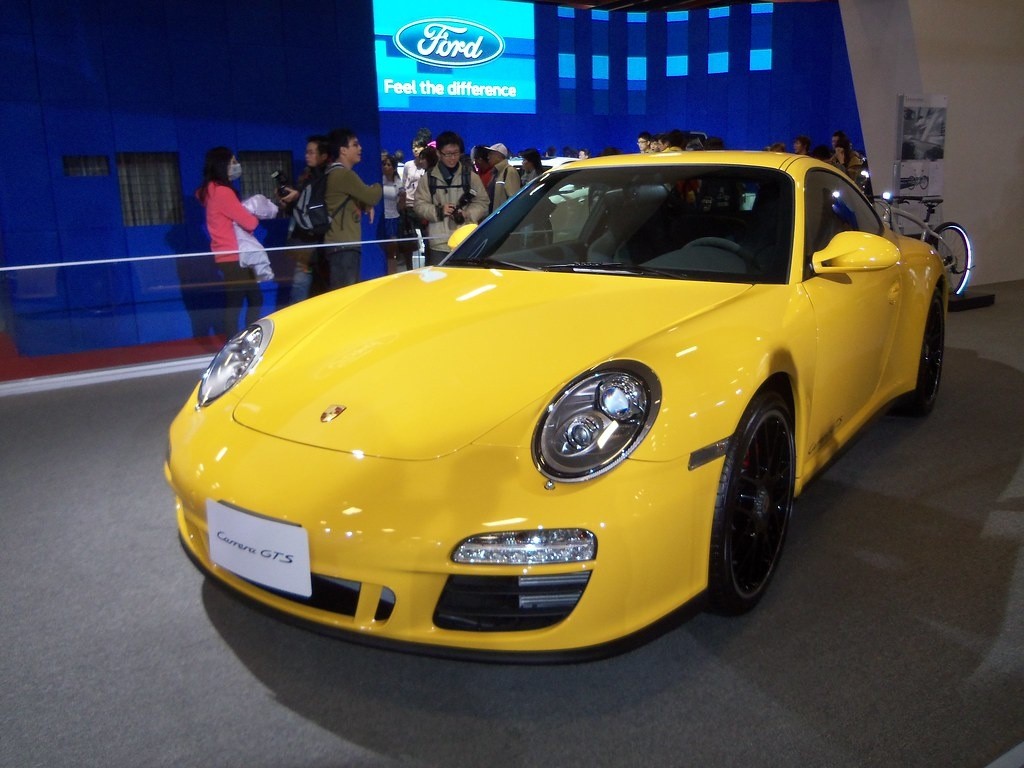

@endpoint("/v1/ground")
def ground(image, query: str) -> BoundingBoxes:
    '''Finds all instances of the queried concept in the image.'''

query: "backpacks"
[292,162,350,242]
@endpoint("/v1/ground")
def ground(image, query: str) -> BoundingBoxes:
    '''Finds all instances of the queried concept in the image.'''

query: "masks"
[228,164,243,180]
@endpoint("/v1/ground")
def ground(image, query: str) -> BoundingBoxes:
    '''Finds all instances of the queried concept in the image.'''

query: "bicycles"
[873,192,972,298]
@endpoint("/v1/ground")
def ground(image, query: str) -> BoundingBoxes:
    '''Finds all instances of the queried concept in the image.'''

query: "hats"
[486,142,509,157]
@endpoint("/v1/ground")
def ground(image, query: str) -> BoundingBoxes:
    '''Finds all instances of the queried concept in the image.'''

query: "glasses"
[637,141,647,145]
[440,148,460,157]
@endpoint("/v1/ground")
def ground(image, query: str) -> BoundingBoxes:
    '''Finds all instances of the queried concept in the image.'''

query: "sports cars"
[165,148,950,665]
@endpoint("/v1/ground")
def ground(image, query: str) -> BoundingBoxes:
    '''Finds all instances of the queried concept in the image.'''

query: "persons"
[275,127,862,306]
[195,147,263,341]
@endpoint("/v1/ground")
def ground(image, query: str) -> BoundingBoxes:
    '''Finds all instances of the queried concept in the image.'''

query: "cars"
[506,157,665,245]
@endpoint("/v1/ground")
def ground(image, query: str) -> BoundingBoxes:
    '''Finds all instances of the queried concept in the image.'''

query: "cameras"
[271,171,294,198]
[449,190,477,225]
